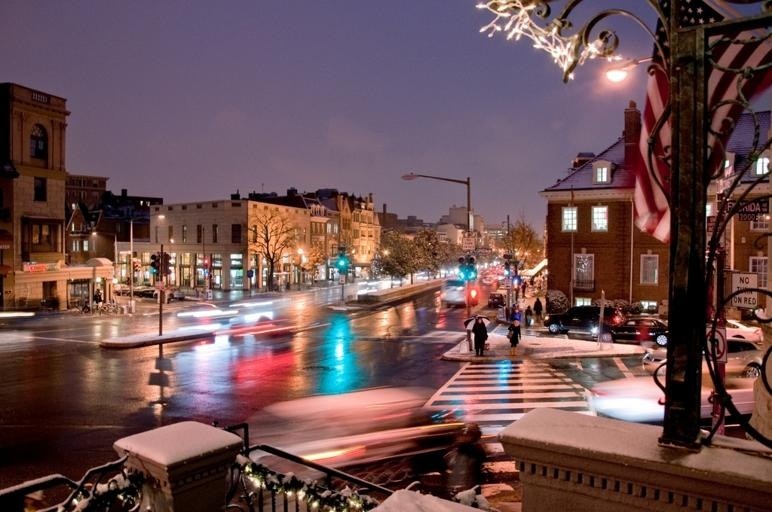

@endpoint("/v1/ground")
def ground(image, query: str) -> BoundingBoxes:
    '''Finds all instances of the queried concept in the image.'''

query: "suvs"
[488,292,505,309]
[247,388,484,498]
[544,307,623,338]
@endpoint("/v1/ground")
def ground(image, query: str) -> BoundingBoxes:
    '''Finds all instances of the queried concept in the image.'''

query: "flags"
[632,0,772,248]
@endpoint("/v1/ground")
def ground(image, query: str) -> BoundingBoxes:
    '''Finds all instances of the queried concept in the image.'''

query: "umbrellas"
[462,313,492,330]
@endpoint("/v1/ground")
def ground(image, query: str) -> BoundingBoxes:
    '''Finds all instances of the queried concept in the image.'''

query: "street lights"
[605,56,654,84]
[402,171,472,236]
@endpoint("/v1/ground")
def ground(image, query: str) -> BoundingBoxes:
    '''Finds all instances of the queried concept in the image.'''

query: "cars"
[643,339,766,374]
[153,288,185,301]
[588,376,759,423]
[178,304,236,323]
[706,319,763,343]
[439,277,477,307]
[115,284,156,298]
[591,318,668,345]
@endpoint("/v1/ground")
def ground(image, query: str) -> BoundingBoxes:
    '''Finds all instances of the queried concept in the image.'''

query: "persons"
[520,282,528,299]
[93,288,103,317]
[642,349,655,363]
[437,422,486,510]
[757,304,768,334]
[471,316,488,357]
[507,318,521,356]
[510,297,543,331]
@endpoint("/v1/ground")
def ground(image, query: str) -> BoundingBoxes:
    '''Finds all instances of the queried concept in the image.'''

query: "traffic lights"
[164,254,171,275]
[503,261,509,276]
[338,253,346,267]
[149,253,159,269]
[458,256,476,281]
